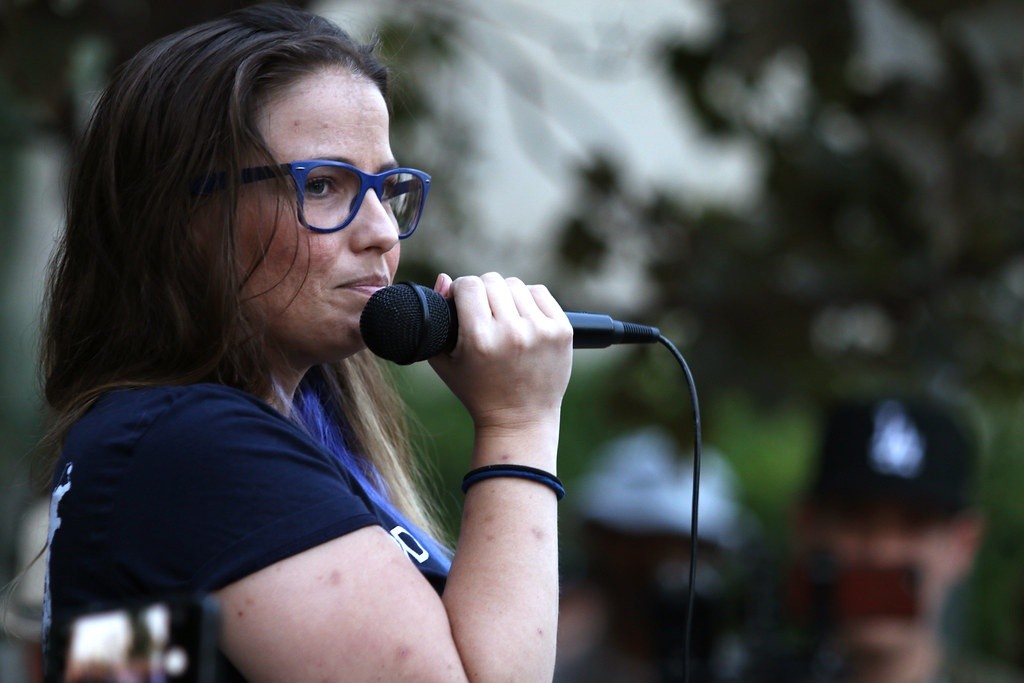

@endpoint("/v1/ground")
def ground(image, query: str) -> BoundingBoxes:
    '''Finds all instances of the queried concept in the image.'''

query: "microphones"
[357,280,658,365]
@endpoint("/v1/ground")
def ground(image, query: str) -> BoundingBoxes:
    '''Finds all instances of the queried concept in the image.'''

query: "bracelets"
[461,464,565,500]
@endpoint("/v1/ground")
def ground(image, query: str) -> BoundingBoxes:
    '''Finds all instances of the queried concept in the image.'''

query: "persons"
[38,4,572,683]
[554,396,1008,683]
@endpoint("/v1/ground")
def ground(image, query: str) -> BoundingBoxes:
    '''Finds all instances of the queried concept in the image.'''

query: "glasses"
[191,160,430,239]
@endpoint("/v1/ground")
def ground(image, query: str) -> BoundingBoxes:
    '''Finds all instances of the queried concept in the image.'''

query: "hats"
[575,420,741,545]
[802,387,979,528]
